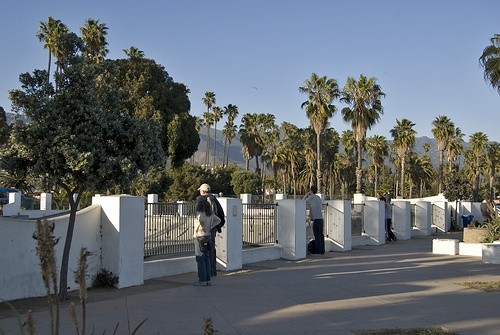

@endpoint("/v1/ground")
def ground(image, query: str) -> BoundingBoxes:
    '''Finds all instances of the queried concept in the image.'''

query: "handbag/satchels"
[199,237,212,252]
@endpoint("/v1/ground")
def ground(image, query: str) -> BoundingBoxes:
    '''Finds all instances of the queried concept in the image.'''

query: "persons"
[376,192,399,243]
[198,184,217,277]
[303,187,326,255]
[193,196,221,285]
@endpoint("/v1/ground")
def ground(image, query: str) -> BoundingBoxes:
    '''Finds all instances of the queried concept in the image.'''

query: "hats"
[198,184,211,191]
[196,196,207,201]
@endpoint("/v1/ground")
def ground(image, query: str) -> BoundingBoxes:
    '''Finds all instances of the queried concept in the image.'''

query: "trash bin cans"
[461,214,474,227]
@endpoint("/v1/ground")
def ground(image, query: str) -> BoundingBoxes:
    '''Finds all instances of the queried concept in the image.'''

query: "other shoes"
[207,280,211,285]
[194,281,207,286]
